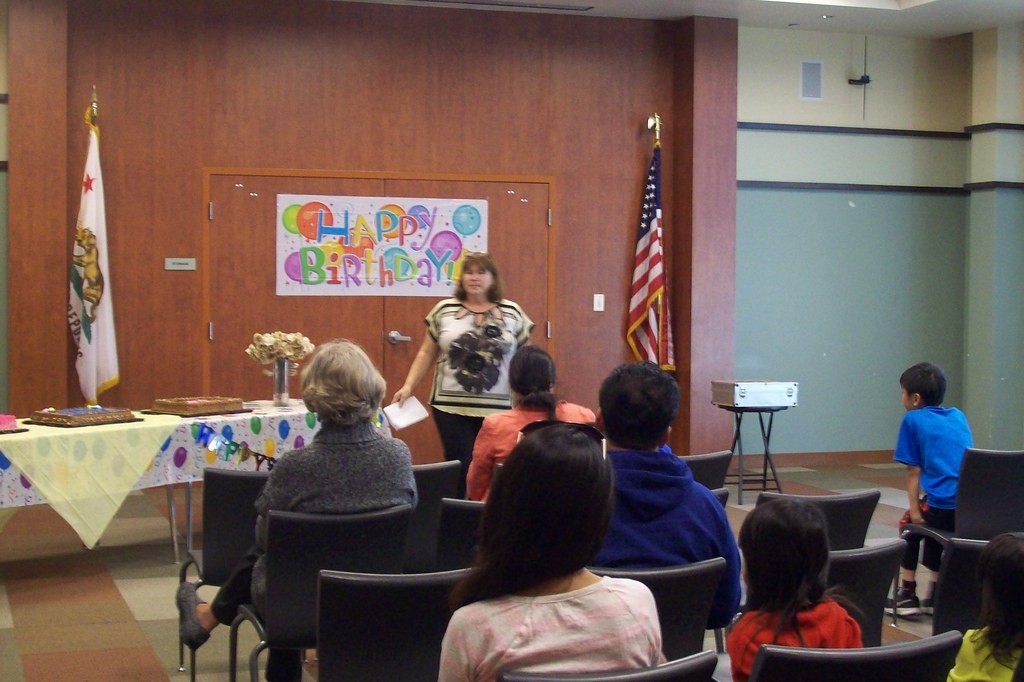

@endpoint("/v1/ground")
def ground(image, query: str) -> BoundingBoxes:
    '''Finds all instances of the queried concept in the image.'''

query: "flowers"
[246,329,317,366]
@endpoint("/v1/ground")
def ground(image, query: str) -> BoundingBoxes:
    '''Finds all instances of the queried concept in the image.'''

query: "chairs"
[739,446,1024,682]
[203,459,490,682]
[495,448,733,682]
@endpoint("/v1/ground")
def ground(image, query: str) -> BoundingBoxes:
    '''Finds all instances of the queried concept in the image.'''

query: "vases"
[274,358,289,407]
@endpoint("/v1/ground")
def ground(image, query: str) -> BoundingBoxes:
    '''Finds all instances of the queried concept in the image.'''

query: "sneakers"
[884,596,922,617]
[919,598,934,615]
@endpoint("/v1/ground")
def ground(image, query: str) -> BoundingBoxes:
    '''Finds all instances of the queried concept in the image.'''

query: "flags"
[626,138,677,372]
[68,93,120,409]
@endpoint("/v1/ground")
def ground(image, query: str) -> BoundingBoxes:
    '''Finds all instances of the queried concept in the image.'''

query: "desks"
[716,405,784,504]
[0,400,392,566]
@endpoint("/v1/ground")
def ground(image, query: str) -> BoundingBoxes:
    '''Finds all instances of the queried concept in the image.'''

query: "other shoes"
[176,582,211,651]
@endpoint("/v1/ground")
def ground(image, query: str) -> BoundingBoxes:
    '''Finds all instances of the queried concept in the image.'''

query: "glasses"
[463,250,490,259]
[515,418,608,462]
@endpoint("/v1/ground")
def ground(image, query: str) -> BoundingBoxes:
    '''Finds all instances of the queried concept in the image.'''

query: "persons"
[726,499,864,682]
[582,362,741,630]
[946,530,1024,682]
[467,346,596,504]
[391,252,538,466]
[176,338,419,682]
[437,420,666,682]
[882,362,976,617]
